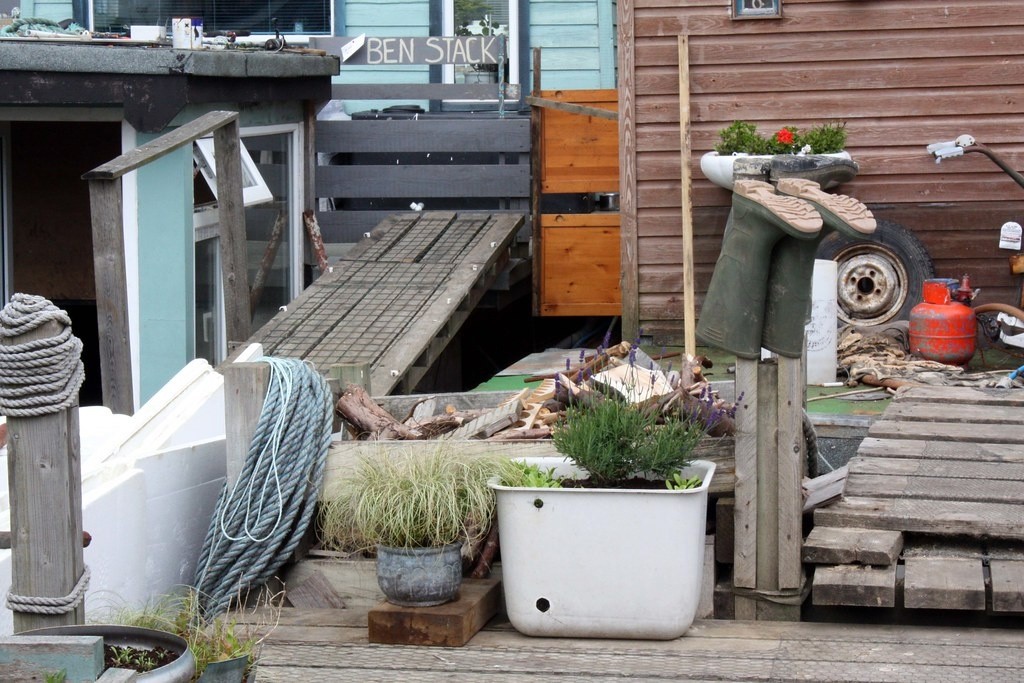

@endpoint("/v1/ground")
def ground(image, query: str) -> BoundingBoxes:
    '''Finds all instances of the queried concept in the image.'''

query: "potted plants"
[488,453,716,640]
[315,429,502,607]
[8,584,290,683]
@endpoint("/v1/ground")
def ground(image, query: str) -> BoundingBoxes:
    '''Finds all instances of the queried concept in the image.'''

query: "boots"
[695,178,824,360]
[762,178,879,359]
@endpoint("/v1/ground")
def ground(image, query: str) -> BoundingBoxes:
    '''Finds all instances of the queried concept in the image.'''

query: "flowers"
[550,318,746,486]
[715,116,850,157]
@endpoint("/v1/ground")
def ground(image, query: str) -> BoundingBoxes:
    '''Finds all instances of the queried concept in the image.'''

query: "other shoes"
[769,150,859,194]
[733,153,796,183]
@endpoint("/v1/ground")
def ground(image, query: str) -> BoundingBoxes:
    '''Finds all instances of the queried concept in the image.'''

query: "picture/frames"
[732,0,783,20]
